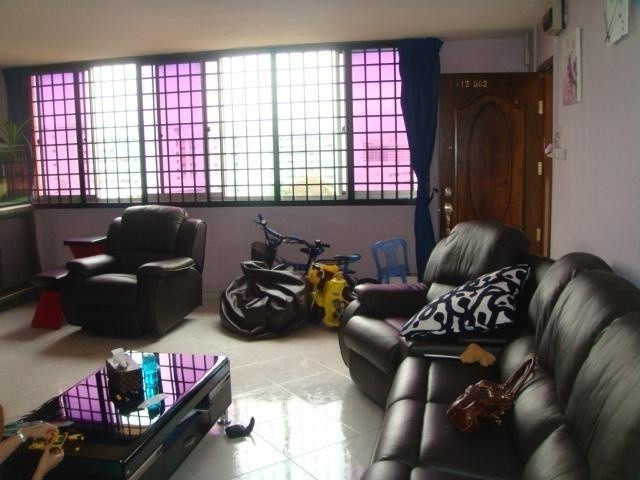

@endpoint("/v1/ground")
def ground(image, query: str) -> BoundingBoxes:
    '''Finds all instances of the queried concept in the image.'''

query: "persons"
[0,404,65,480]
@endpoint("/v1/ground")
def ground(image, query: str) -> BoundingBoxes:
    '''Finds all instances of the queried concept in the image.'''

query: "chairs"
[373,238,410,286]
[60,204,208,339]
[339,220,556,408]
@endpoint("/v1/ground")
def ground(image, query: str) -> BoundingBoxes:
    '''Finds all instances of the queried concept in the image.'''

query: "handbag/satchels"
[445,380,513,432]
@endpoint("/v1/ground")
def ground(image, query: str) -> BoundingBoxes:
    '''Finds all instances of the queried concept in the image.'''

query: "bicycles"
[248,213,379,287]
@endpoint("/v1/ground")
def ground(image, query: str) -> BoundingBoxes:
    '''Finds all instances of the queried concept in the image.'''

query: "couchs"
[362,252,639,480]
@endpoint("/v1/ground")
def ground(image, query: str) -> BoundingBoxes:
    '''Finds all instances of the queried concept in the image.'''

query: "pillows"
[399,263,534,340]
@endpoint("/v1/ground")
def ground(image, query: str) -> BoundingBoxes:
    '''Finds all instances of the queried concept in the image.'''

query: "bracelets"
[16,431,27,443]
